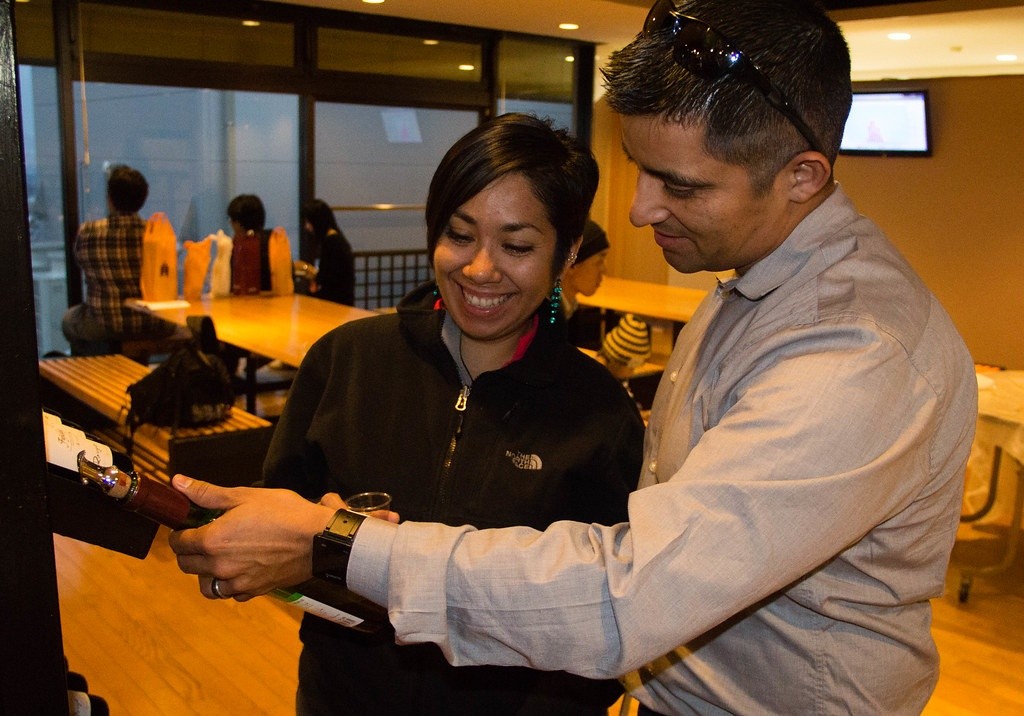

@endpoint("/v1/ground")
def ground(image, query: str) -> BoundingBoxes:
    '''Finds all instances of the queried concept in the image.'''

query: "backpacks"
[120,315,236,458]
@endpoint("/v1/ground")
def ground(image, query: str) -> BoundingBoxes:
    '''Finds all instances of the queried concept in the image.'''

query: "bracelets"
[301,263,308,275]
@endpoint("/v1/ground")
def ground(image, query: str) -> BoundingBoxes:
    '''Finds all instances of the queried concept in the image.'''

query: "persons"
[73,166,150,352]
[169,0,978,716]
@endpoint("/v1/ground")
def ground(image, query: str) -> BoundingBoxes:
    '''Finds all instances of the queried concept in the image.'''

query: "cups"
[345,492,392,522]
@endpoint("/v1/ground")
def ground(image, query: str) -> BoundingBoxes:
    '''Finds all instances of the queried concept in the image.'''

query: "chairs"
[951,370,1023,604]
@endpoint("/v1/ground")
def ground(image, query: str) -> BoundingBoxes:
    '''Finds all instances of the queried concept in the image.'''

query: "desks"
[122,291,381,416]
[575,274,708,351]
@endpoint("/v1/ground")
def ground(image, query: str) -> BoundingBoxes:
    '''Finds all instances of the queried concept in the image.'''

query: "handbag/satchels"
[182,235,211,300]
[270,225,295,295]
[138,213,178,301]
[211,225,233,298]
[231,229,261,296]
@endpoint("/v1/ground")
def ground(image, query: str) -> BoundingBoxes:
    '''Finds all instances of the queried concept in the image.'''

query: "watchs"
[311,508,367,595]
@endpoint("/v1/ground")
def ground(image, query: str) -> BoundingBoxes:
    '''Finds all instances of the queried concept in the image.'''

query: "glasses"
[643,0,828,160]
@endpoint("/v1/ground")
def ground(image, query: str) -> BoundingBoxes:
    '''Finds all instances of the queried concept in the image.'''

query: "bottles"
[75,450,387,634]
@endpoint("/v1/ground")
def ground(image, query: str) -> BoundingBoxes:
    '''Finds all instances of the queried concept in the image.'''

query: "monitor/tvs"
[838,89,932,156]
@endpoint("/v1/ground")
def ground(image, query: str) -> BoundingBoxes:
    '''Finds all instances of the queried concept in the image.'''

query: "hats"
[603,313,652,362]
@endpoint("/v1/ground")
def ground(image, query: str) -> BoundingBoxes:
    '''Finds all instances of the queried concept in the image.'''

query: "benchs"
[37,356,272,487]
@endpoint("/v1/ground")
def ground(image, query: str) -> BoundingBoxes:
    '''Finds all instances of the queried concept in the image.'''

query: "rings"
[211,578,226,599]
